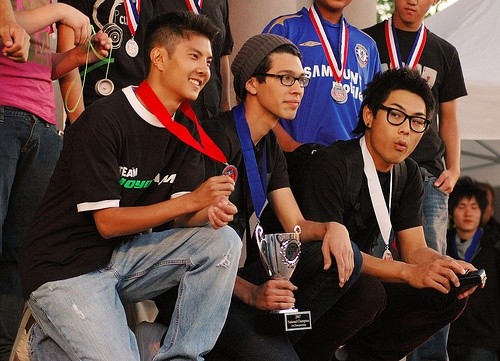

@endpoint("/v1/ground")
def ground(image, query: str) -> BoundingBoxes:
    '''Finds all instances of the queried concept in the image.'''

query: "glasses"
[254,71,310,88]
[374,103,431,133]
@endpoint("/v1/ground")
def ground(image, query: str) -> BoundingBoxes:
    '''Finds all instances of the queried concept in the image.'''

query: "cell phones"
[450,268,487,288]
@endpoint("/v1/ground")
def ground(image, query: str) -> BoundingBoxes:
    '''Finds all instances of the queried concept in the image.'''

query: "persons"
[0,0,112,361]
[360,0,468,254]
[447,178,500,292]
[10,9,243,360]
[198,34,360,358]
[149,0,234,148]
[302,69,470,361]
[253,0,381,181]
[57,0,145,149]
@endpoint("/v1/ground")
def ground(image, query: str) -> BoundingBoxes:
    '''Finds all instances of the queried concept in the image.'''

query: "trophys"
[254,223,312,331]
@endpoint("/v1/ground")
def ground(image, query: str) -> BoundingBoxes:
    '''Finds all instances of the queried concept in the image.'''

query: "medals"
[382,250,393,260]
[330,84,348,103]
[222,164,238,183]
[125,38,139,57]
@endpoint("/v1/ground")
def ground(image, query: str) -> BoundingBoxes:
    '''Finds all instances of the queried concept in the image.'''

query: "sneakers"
[136,320,167,361]
[9,306,37,361]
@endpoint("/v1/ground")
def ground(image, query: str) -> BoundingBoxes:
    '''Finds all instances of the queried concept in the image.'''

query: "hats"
[231,34,303,84]
